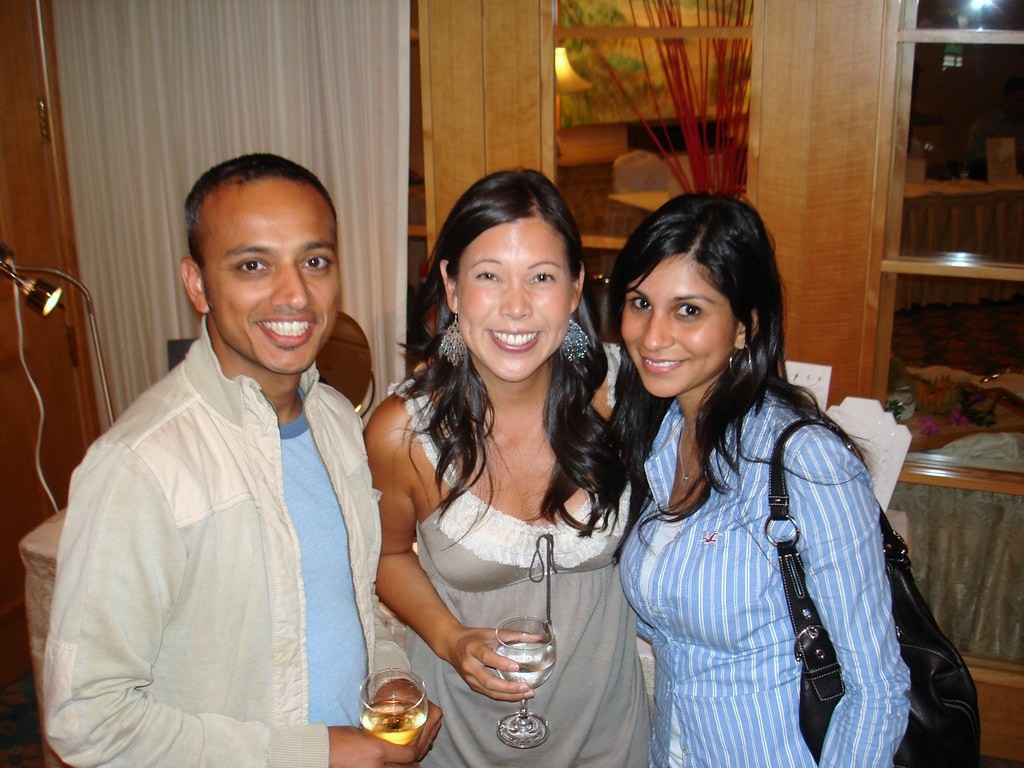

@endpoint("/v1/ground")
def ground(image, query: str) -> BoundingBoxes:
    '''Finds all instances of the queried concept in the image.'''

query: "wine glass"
[493,616,557,750]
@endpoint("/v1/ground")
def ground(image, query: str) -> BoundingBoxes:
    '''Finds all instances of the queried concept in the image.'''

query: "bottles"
[407,263,439,356]
[886,350,915,425]
[925,44,978,179]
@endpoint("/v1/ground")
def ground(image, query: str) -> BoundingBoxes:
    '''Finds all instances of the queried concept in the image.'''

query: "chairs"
[613,148,720,193]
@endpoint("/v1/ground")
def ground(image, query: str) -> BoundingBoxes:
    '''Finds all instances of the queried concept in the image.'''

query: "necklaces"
[679,430,697,487]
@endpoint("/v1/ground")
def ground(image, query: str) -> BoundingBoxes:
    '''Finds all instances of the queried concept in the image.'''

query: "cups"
[359,668,429,746]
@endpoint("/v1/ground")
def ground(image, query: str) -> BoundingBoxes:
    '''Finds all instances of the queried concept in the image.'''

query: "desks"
[883,365,1024,667]
[609,174,1024,311]
[20,504,657,768]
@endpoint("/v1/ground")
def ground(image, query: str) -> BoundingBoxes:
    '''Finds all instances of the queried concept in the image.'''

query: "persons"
[363,168,652,768]
[43,154,443,768]
[605,192,910,768]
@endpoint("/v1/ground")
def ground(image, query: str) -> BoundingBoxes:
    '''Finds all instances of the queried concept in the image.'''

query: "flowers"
[918,390,1001,437]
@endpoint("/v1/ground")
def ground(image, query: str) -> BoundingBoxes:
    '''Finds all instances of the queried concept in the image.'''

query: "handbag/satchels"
[763,419,981,768]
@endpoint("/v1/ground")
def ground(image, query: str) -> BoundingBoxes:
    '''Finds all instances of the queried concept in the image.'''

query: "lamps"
[554,47,593,94]
[0,243,114,426]
